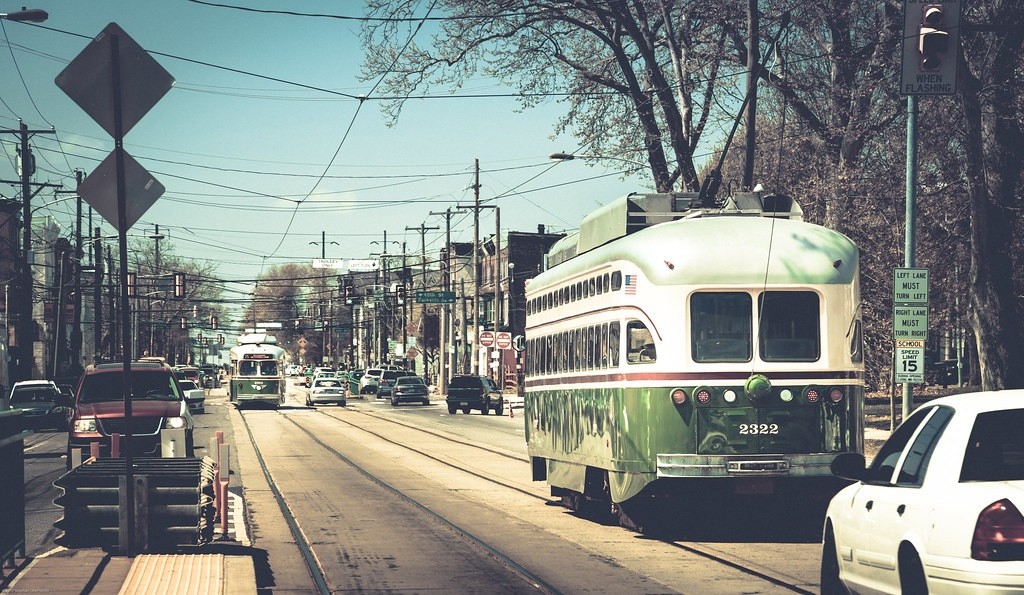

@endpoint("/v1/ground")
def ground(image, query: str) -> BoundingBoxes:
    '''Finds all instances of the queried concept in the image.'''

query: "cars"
[8,379,76,433]
[305,377,346,408]
[816,390,1024,595]
[283,362,416,399]
[172,363,226,415]
[390,376,429,407]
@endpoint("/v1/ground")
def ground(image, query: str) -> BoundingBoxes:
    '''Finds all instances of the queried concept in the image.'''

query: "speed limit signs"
[894,349,926,384]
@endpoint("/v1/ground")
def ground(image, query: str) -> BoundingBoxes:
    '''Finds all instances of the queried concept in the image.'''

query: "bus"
[514,185,871,535]
[226,332,290,408]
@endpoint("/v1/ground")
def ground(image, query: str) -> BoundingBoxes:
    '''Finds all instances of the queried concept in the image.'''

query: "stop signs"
[480,332,495,347]
[497,333,511,348]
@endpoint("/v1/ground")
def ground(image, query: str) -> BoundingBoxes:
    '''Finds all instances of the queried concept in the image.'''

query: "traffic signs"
[415,291,458,304]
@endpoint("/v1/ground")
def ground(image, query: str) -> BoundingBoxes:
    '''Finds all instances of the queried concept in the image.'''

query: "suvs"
[445,373,504,418]
[57,357,206,471]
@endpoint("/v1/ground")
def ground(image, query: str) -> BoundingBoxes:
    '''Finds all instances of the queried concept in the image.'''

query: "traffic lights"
[900,0,961,96]
